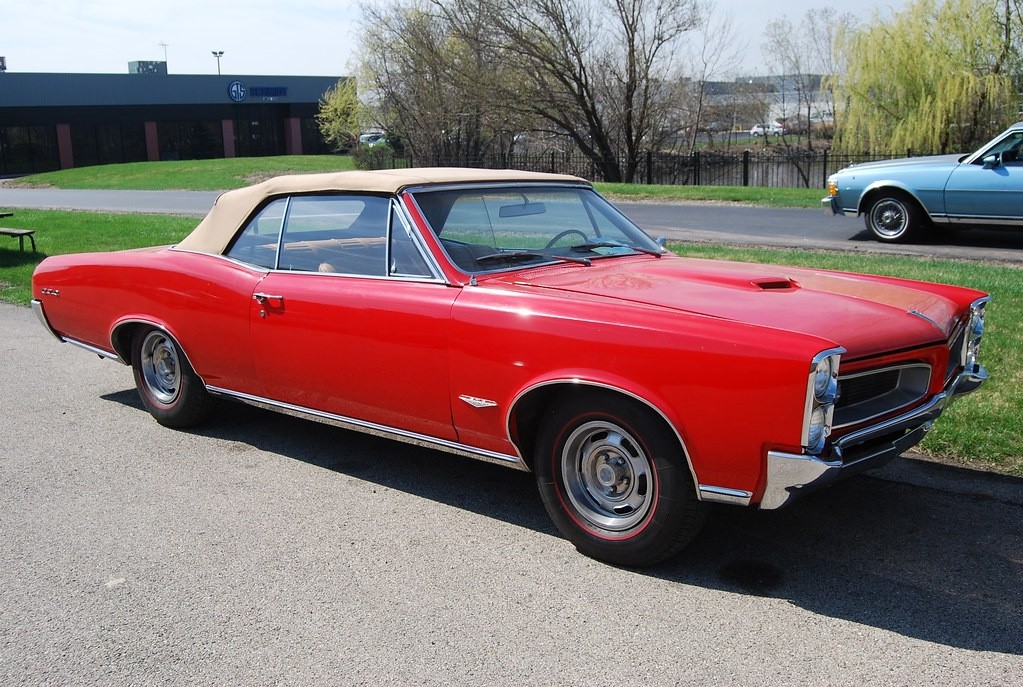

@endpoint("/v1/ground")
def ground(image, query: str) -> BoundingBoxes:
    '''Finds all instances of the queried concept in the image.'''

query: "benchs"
[0,228,37,257]
[237,235,400,270]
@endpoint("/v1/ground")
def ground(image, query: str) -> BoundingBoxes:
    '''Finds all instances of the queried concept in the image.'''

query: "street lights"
[212,52,224,75]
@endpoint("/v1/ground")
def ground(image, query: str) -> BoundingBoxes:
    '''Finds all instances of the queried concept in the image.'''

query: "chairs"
[317,257,384,275]
[442,242,509,272]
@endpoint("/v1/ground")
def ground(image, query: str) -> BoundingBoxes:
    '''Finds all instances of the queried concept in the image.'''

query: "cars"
[819,121,1023,245]
[750,124,787,137]
[31,167,991,566]
